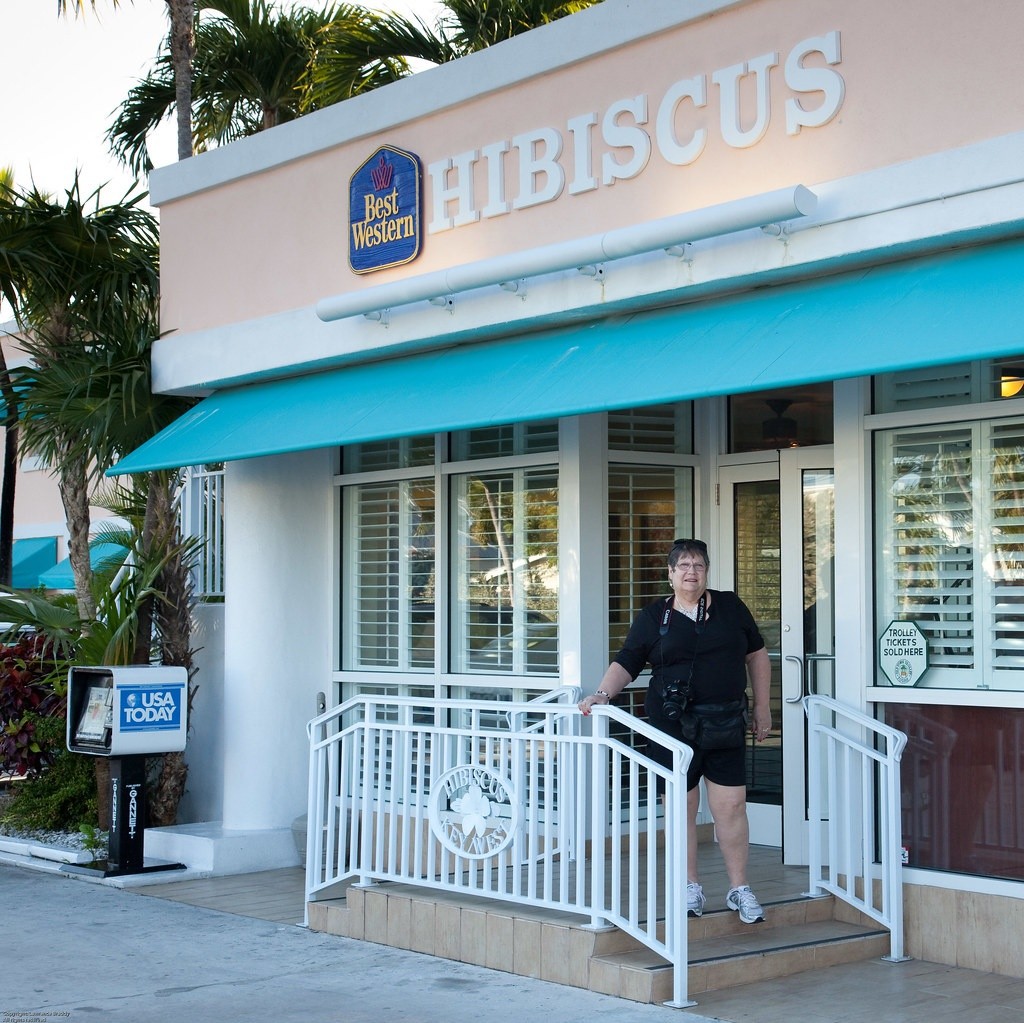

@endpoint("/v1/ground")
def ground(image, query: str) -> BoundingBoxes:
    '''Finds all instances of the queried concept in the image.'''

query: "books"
[73,687,115,743]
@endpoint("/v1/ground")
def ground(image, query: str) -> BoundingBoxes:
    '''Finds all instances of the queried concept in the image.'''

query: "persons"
[577,539,772,928]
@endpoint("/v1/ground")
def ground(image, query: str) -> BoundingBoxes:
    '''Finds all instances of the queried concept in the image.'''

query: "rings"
[763,728,768,732]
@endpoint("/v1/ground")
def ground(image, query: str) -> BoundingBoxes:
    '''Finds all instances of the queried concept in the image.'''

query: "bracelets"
[595,690,610,702]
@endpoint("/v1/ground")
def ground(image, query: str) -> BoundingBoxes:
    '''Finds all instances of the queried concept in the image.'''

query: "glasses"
[674,560,708,571]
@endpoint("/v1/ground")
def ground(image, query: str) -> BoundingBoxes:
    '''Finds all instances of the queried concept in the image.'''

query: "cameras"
[660,679,694,719]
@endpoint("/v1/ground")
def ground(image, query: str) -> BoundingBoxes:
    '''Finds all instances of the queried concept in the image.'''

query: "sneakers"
[726,886,766,924]
[686,883,706,917]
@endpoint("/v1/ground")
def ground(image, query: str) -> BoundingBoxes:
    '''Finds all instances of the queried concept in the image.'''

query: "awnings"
[10,536,58,590]
[104,231,1024,477]
[37,531,136,590]
